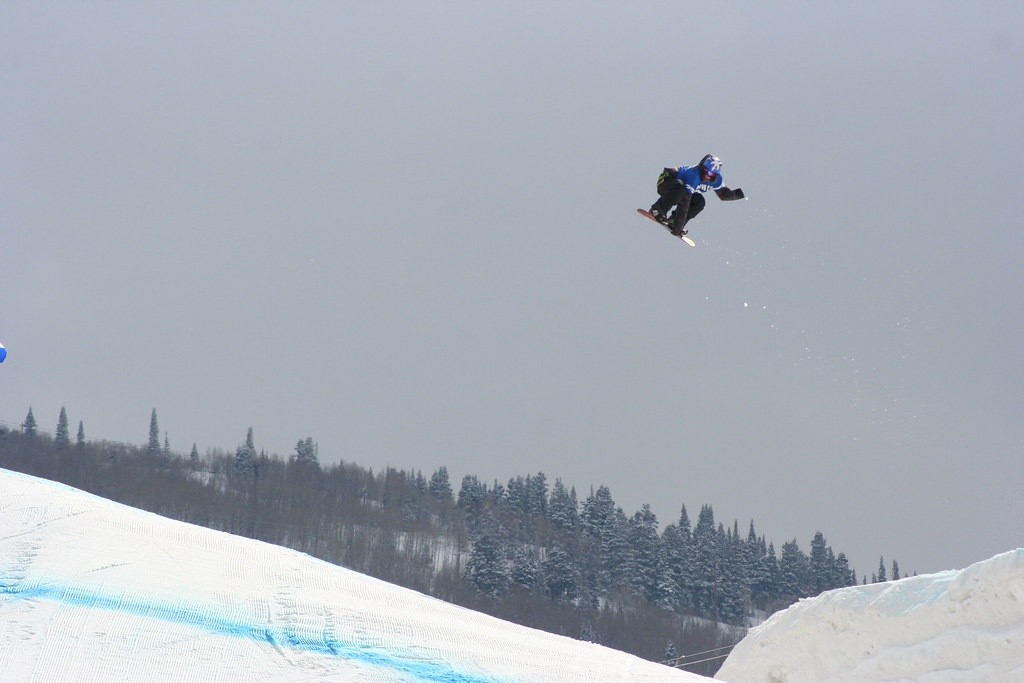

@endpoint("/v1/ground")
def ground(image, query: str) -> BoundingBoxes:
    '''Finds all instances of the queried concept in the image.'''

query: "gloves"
[734,188,744,199]
[671,222,684,236]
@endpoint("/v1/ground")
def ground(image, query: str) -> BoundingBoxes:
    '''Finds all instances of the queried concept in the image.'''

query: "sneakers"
[650,208,668,225]
[667,216,688,235]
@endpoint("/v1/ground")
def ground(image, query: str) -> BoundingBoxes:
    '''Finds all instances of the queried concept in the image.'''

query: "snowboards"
[636,208,696,247]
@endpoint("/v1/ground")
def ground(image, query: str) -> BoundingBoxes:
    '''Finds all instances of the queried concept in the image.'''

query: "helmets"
[703,156,721,174]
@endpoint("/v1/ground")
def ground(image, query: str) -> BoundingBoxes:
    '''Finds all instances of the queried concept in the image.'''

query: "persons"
[649,153,744,236]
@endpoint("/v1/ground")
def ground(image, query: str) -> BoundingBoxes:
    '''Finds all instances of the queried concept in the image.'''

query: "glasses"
[703,168,714,178]
